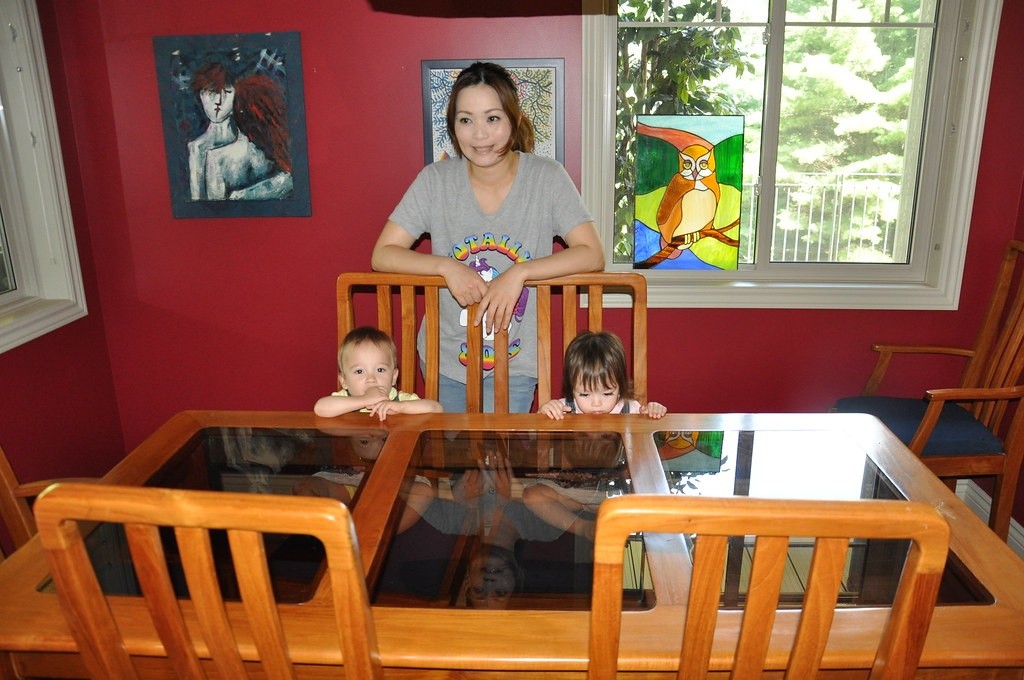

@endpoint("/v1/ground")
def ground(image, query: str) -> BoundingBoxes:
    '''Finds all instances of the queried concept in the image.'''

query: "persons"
[371,62,607,414]
[524,331,668,549]
[397,431,635,609]
[294,326,445,536]
[285,430,432,533]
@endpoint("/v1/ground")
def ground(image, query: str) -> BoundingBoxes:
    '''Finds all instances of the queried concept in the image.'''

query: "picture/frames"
[418,57,567,167]
[152,31,312,220]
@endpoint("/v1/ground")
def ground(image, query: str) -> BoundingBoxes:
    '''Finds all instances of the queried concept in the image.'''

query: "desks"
[0,405,1024,680]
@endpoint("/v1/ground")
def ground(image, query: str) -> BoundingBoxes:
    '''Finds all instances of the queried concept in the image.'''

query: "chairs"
[326,427,485,482]
[30,478,384,680]
[494,429,633,500]
[0,444,59,560]
[829,235,1024,549]
[335,273,484,415]
[493,272,648,417]
[579,488,955,680]
